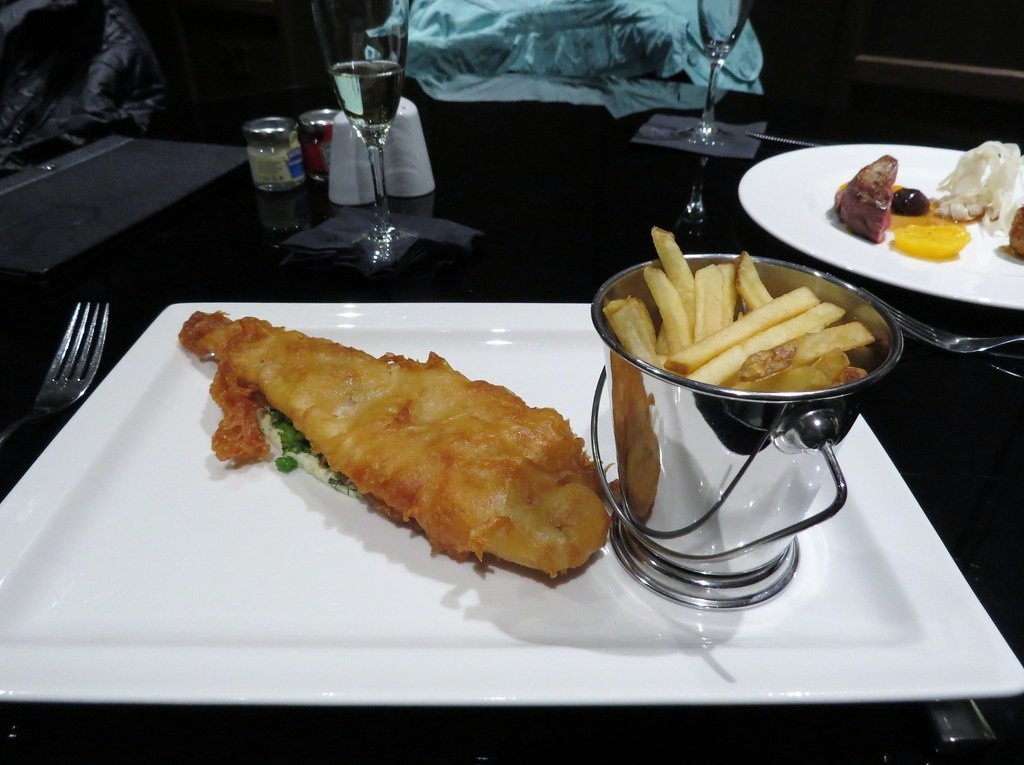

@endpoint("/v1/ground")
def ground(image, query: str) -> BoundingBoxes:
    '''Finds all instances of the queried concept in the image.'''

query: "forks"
[0,302,111,446]
[856,287,1024,354]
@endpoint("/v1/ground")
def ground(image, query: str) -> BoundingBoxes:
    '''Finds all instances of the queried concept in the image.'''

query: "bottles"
[244,118,303,190]
[301,110,350,179]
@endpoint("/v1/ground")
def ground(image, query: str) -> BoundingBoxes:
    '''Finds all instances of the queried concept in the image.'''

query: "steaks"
[839,156,899,243]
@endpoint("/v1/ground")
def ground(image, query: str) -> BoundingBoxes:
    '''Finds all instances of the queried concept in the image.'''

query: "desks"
[0,87,1024,765]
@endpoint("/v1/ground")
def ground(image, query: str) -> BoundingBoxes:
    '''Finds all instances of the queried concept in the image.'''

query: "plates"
[0,302,1024,706]
[740,143,1024,309]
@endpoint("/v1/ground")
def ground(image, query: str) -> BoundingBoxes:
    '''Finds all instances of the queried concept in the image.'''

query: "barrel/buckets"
[593,256,903,609]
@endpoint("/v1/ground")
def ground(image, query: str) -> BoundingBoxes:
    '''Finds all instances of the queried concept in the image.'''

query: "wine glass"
[309,0,419,262]
[676,0,753,145]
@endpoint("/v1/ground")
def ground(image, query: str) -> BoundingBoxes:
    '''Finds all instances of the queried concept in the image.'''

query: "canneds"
[243,109,340,192]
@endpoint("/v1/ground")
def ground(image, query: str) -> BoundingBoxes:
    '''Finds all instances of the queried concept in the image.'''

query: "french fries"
[600,226,876,395]
